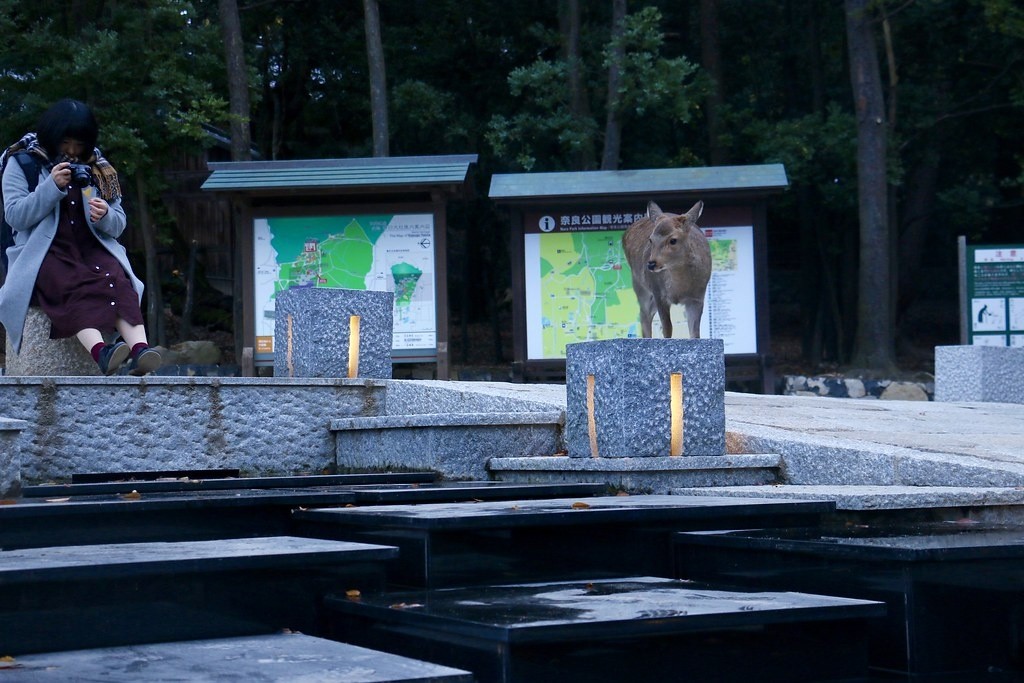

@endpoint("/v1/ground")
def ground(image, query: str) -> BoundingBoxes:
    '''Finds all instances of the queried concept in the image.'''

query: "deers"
[622,199,712,339]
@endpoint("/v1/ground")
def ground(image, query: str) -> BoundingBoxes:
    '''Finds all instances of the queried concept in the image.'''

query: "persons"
[1,99,162,377]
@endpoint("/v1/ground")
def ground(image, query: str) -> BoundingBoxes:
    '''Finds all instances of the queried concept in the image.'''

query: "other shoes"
[98,341,130,376]
[129,348,161,376]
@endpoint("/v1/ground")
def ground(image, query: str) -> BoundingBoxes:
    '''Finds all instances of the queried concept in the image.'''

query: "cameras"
[67,164,91,188]
